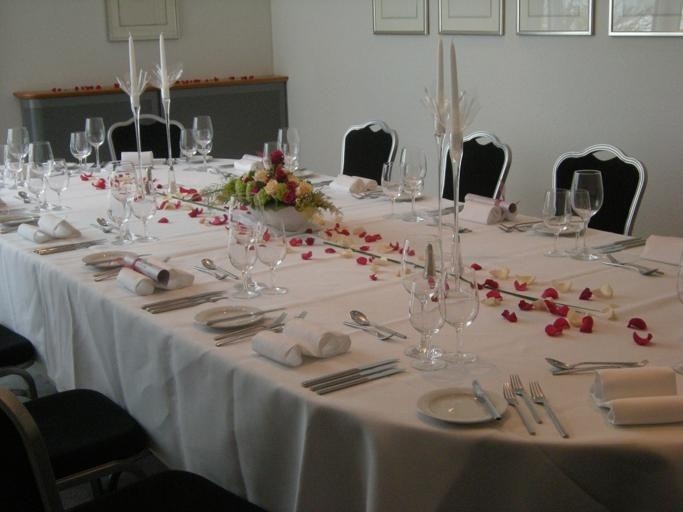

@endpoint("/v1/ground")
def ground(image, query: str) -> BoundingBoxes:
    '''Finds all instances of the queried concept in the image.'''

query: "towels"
[589,366,683,426]
[17,213,195,295]
[233,154,264,172]
[641,234,683,266]
[252,318,352,367]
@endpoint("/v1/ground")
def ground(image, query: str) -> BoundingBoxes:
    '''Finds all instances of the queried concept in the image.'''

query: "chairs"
[107,113,187,161]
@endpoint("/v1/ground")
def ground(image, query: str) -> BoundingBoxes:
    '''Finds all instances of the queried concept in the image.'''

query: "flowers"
[187,150,343,220]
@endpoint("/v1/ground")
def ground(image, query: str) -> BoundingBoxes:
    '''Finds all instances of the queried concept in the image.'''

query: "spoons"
[542,357,638,367]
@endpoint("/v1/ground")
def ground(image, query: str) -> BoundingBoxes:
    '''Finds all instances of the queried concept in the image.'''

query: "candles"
[160,31,169,99]
[434,38,462,132]
[128,32,139,104]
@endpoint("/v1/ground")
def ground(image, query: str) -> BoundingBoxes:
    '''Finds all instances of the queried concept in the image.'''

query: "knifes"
[471,380,503,422]
[301,357,403,395]
[593,231,667,278]
[551,365,641,376]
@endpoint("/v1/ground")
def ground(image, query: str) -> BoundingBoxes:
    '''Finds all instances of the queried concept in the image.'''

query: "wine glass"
[0,114,605,374]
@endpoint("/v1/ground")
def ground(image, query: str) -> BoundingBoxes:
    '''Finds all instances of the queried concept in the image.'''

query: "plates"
[415,387,509,424]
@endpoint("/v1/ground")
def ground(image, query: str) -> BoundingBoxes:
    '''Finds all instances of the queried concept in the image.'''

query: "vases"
[244,203,319,232]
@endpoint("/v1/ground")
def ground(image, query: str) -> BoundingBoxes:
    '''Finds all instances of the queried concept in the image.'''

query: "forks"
[527,380,569,439]
[507,374,543,424]
[501,383,537,434]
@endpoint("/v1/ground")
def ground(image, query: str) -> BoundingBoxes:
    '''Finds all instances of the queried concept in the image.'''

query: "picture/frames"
[104,0,182,43]
